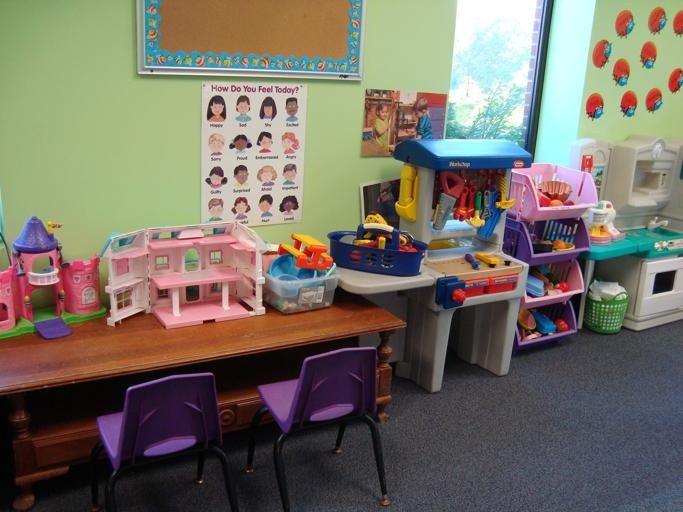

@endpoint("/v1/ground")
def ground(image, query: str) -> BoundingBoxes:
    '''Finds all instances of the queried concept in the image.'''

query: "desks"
[0,288,409,509]
[577,227,682,331]
[335,240,529,393]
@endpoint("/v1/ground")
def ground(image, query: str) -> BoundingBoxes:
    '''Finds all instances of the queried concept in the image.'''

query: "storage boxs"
[262,269,341,314]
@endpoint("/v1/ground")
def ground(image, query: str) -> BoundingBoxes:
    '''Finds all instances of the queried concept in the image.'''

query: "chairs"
[90,371,238,511]
[246,347,387,512]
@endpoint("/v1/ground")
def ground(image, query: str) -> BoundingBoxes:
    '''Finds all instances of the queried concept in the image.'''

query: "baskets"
[583,291,630,334]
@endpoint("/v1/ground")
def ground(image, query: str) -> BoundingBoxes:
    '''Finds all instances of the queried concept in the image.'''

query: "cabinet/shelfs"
[503,163,597,351]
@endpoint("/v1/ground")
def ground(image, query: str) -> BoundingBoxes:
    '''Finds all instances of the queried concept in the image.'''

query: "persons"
[369,101,394,155]
[410,96,434,141]
